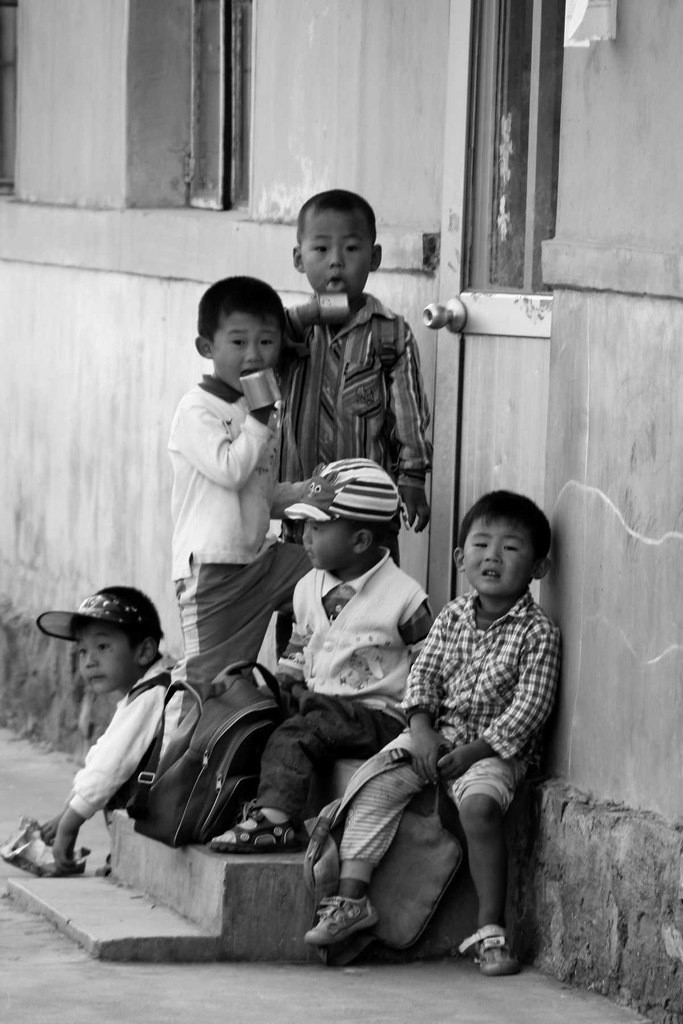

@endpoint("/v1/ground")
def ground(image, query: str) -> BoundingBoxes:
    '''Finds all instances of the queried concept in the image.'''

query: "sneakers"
[305,896,380,945]
[458,924,520,975]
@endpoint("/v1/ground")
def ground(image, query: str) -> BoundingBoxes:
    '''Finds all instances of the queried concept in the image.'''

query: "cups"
[239,368,281,411]
[312,293,350,325]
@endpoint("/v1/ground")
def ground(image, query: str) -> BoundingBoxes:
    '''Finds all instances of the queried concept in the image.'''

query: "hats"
[36,586,159,641]
[284,458,399,522]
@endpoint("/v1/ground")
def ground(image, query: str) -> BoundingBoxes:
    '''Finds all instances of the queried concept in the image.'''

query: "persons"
[36,586,181,876]
[275,190,434,661]
[303,489,560,976]
[207,457,434,854]
[150,275,315,787]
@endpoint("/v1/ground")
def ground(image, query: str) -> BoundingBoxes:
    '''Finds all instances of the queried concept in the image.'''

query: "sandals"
[206,810,309,854]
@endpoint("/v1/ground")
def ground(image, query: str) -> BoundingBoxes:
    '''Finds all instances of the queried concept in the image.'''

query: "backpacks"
[127,660,284,849]
[305,748,464,967]
